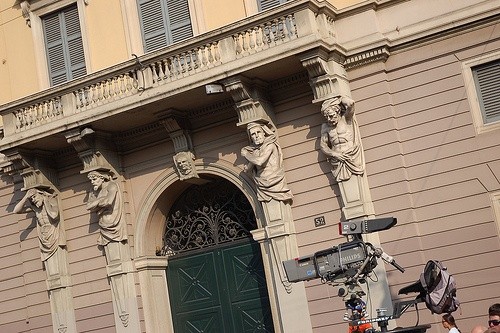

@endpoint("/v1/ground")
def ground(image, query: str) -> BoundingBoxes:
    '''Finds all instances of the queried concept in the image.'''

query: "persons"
[85,171,129,247]
[319,95,364,184]
[174,152,192,175]
[12,188,67,263]
[471,303,500,333]
[241,122,294,203]
[441,315,462,333]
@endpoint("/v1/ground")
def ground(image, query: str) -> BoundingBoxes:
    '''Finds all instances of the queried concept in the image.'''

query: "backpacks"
[419,259,462,316]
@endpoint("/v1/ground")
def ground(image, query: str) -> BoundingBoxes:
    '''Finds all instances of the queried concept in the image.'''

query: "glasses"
[488,319,500,326]
[442,320,447,324]
